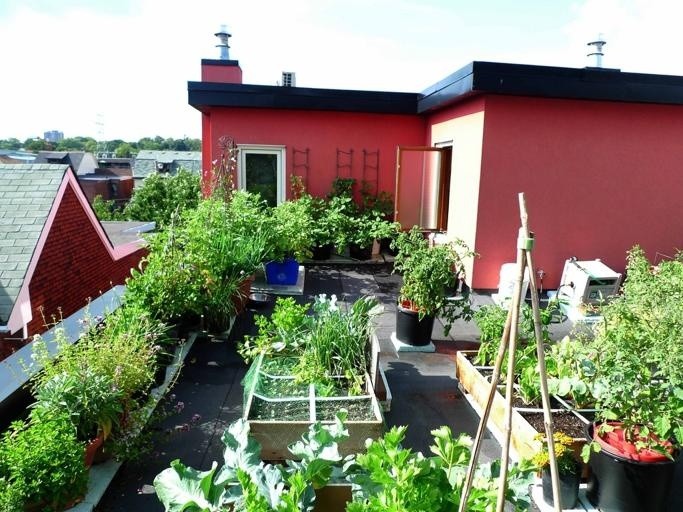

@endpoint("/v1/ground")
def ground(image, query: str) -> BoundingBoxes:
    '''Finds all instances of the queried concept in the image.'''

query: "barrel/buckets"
[542,458,582,508]
[313,236,397,260]
[395,300,434,345]
[497,262,529,308]
[584,418,673,512]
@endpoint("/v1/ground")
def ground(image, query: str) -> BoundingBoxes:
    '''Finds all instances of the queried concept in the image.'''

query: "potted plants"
[0,134,683,512]
